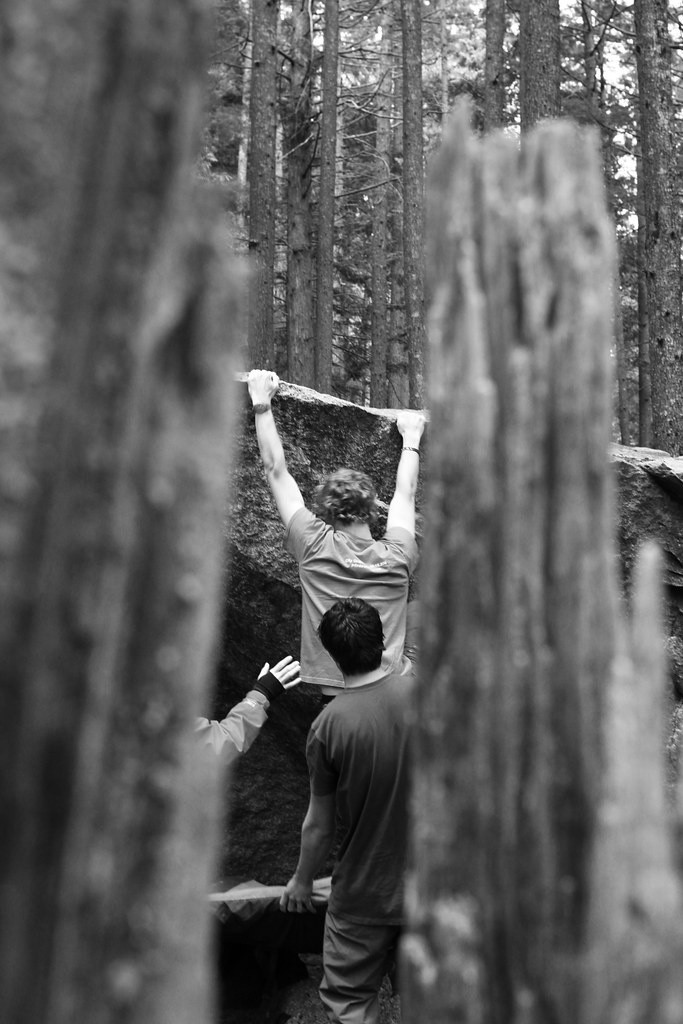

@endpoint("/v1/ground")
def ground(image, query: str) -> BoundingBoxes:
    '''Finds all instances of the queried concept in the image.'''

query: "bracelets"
[400,446,421,457]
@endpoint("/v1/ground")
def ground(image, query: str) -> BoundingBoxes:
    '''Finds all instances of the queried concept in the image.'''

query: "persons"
[279,598,415,1023]
[246,369,426,715]
[190,654,302,782]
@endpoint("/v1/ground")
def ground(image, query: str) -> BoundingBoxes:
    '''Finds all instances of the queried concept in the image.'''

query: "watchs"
[251,402,272,414]
